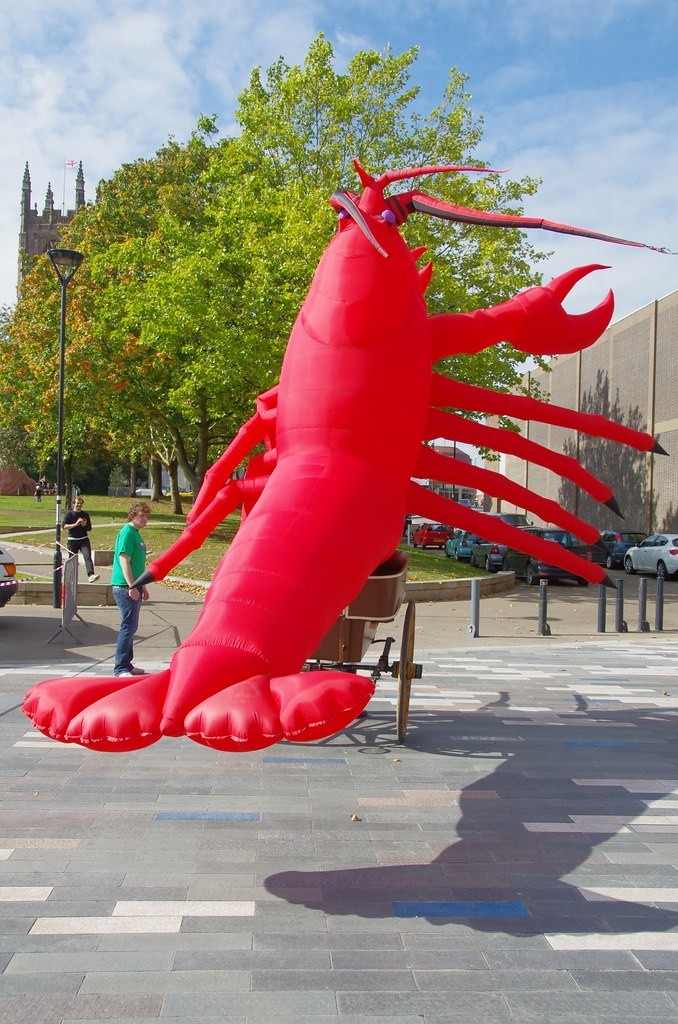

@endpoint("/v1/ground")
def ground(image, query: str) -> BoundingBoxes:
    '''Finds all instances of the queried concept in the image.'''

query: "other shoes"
[119,672,133,677]
[130,668,144,675]
[89,574,100,583]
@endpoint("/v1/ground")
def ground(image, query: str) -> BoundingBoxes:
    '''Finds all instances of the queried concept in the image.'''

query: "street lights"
[47,249,84,609]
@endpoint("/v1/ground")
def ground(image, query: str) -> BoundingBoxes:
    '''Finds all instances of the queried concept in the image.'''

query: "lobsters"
[21,162,676,755]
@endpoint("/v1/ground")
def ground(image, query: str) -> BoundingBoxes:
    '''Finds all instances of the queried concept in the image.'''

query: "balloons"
[22,154,677,753]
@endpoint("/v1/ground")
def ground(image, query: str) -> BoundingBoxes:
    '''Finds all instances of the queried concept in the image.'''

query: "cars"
[503,549,588,588]
[471,538,507,572]
[458,499,471,507]
[0,547,20,606]
[412,523,452,550]
[623,534,678,581]
[497,514,649,570]
[444,530,480,561]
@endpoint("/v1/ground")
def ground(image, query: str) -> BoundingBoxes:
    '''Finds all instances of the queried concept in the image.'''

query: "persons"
[34,485,42,502]
[62,498,101,583]
[40,475,48,489]
[111,502,150,677]
